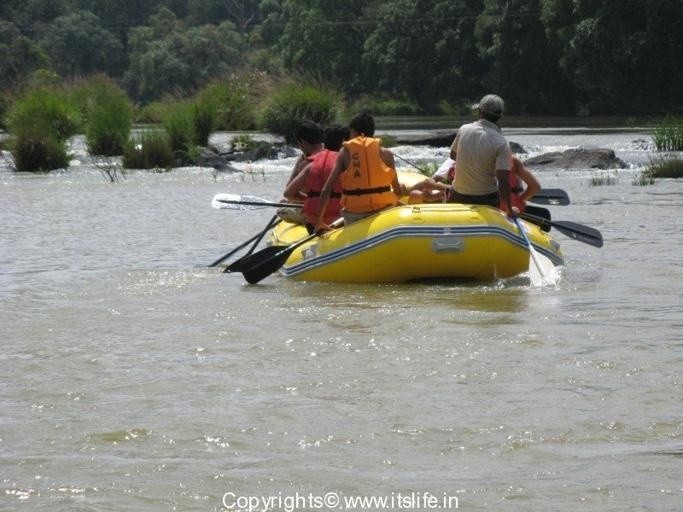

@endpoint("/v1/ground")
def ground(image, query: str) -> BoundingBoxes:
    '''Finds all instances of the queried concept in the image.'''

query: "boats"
[268,166,564,283]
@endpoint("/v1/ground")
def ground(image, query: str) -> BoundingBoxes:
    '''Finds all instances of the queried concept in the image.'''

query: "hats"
[471,94,505,117]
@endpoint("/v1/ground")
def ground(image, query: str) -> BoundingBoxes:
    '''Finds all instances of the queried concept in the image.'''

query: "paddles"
[512,187,603,247]
[512,215,559,288]
[212,193,304,211]
[222,218,344,285]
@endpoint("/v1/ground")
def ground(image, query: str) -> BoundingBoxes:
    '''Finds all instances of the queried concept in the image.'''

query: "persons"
[276,92,540,236]
